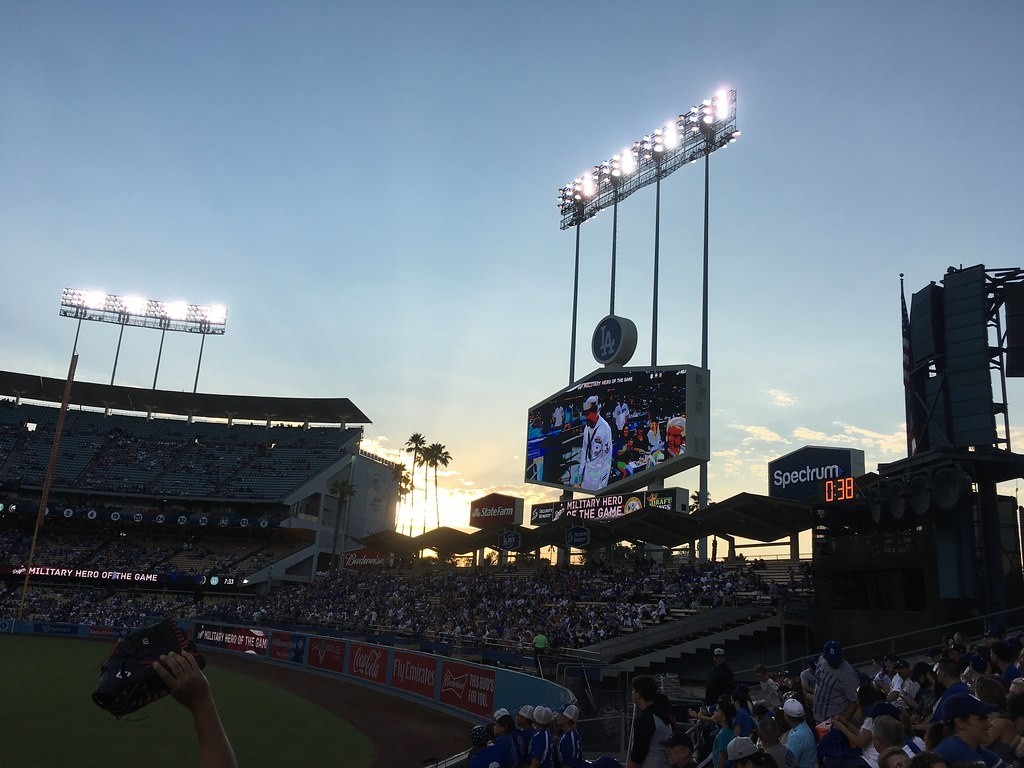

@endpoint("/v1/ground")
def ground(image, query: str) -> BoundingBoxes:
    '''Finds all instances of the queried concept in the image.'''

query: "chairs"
[0,399,363,629]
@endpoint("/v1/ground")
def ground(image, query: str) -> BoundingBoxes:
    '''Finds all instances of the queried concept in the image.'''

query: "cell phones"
[899,695,905,700]
[874,679,878,685]
[700,707,703,712]
[688,709,692,712]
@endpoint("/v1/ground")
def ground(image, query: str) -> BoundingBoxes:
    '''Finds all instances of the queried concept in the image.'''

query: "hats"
[581,395,598,413]
[714,627,1024,768]
[553,703,580,724]
[489,708,509,722]
[585,756,623,768]
[511,704,534,720]
[532,705,553,725]
[659,733,694,751]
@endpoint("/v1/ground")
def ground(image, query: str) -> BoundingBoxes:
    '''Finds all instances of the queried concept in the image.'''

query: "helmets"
[666,416,686,442]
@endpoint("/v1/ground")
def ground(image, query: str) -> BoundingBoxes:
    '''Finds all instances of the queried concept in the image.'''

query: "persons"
[0,494,285,629]
[532,629,548,669]
[624,626,1024,768]
[0,398,332,499]
[467,704,583,768]
[153,650,239,768]
[200,556,814,655]
[530,386,686,491]
